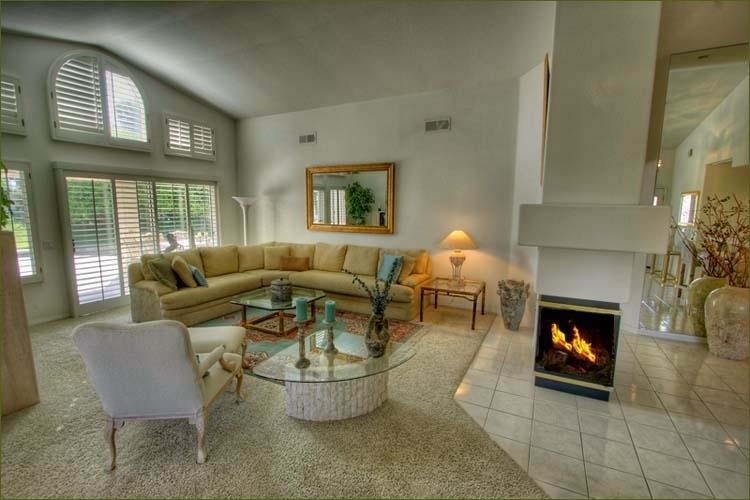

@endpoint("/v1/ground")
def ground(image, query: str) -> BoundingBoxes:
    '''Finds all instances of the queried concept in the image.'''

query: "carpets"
[0,290,567,499]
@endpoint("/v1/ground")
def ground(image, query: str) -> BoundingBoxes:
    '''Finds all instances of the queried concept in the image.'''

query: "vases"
[496,292,528,332]
[681,268,737,341]
[701,278,750,364]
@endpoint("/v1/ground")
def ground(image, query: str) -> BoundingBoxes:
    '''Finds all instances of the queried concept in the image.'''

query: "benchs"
[184,322,249,375]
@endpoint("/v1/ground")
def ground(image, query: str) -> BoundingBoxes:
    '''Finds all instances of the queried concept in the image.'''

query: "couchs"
[124,238,263,330]
[285,238,434,323]
[232,237,317,288]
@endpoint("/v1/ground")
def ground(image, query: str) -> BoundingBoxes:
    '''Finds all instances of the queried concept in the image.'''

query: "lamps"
[231,194,261,246]
[436,227,479,289]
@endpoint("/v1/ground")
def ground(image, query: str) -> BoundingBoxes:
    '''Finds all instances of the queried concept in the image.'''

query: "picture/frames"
[302,157,395,236]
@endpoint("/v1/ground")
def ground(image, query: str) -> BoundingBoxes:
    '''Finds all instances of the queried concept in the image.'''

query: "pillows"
[280,253,310,273]
[262,243,292,271]
[391,248,418,284]
[136,248,211,291]
[375,250,406,286]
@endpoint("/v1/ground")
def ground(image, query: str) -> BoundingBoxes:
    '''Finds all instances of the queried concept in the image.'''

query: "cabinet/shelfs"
[0,222,44,425]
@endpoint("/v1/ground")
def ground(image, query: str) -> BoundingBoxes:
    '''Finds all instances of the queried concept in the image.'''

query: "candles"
[322,299,337,323]
[293,296,310,323]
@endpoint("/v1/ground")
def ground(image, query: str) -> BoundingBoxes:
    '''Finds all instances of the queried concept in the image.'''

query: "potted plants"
[339,254,404,359]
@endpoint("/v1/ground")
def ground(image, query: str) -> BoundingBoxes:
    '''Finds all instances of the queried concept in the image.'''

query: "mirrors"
[675,190,701,228]
[635,41,750,340]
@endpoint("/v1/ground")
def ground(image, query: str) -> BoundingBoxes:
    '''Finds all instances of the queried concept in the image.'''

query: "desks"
[414,273,487,332]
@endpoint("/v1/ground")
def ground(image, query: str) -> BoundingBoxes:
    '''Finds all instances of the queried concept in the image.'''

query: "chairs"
[63,318,244,474]
